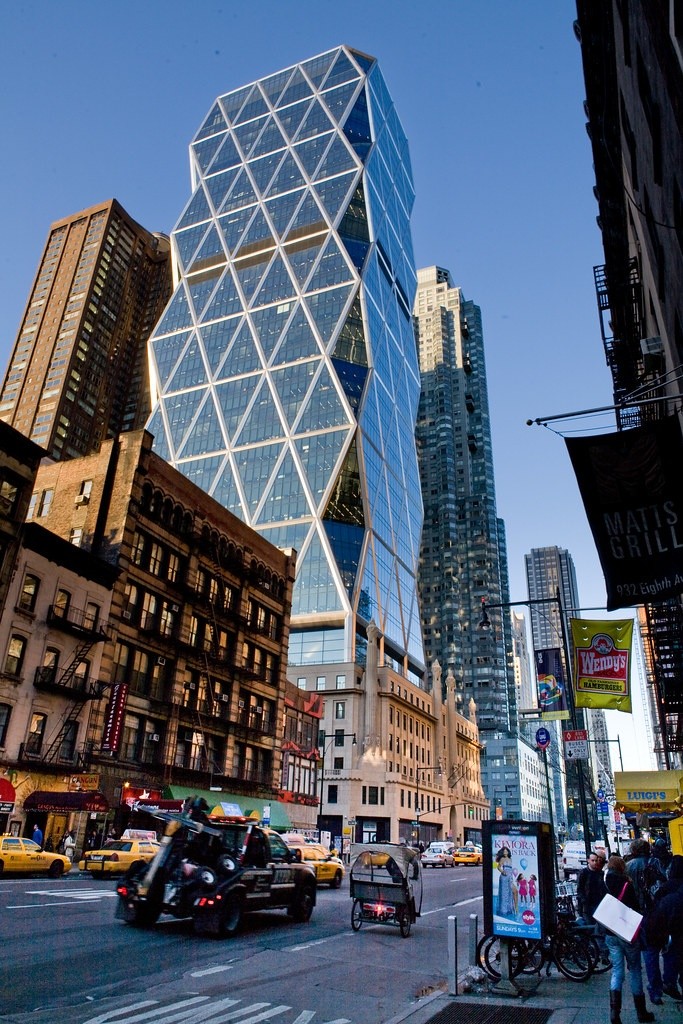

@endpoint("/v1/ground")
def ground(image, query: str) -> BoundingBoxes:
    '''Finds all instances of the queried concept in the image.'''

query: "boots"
[610,990,621,1024]
[633,993,654,1023]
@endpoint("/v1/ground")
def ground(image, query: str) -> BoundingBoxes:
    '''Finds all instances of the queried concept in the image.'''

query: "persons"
[45,827,117,862]
[602,856,654,1024]
[517,874,528,908]
[528,875,537,908]
[496,846,518,915]
[576,836,683,1003]
[419,843,424,853]
[32,825,41,846]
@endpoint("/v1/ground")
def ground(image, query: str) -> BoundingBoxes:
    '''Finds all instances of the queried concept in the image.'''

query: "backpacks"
[642,857,669,903]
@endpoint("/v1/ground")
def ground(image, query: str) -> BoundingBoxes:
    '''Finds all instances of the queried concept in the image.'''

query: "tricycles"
[346,840,426,936]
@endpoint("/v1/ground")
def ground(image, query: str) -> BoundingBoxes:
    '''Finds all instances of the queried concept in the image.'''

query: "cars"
[454,841,483,865]
[420,848,455,868]
[277,832,347,887]
[80,829,165,880]
[0,836,73,876]
[562,839,596,880]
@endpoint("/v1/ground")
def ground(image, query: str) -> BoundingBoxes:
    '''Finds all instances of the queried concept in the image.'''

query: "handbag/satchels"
[593,882,644,944]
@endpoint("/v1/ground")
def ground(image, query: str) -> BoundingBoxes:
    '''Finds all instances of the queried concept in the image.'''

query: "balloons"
[512,868,518,878]
[520,858,527,870]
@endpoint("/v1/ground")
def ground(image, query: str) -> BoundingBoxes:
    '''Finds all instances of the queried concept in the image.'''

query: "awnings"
[614,769,683,820]
[23,791,109,813]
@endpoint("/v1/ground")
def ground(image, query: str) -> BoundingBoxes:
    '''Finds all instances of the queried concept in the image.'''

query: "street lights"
[414,766,444,853]
[483,587,591,867]
[319,733,356,840]
[493,791,513,824]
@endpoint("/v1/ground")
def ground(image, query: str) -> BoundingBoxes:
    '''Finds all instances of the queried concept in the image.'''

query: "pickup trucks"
[116,818,318,933]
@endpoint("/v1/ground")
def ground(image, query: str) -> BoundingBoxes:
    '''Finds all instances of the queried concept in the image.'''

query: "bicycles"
[475,891,617,981]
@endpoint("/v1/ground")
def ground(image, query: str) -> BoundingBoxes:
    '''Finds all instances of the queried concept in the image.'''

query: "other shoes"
[602,959,611,966]
[649,996,662,1005]
[662,983,678,996]
[585,963,599,970]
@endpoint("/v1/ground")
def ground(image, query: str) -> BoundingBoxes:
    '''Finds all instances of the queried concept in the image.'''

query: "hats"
[655,838,666,847]
